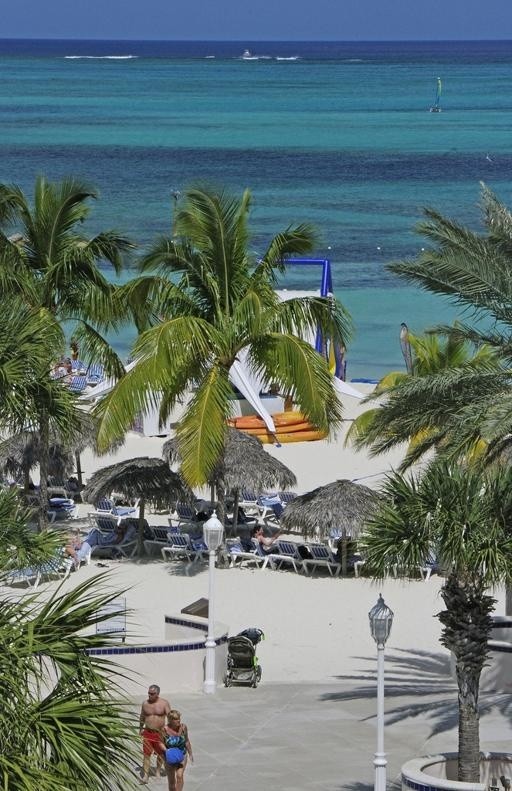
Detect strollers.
[227,627,266,689]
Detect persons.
[338,340,346,381]
[250,525,279,553]
[52,337,79,387]
[112,521,127,545]
[139,685,194,791]
[57,534,82,567]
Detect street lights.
[202,509,226,694]
[369,591,394,791]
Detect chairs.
[88,485,362,582]
[50,360,104,393]
[354,545,391,579]
[392,549,433,581]
[7,485,97,588]
